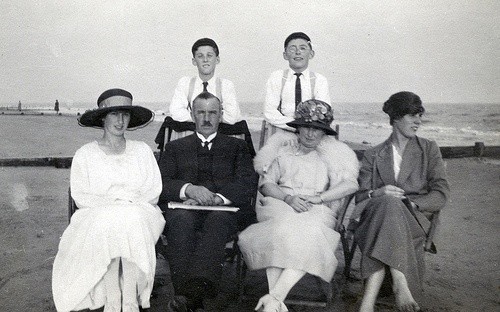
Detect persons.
[18,100,22,111]
[51,88,168,312]
[263,31,333,133]
[353,90,451,312]
[168,38,247,141]
[236,99,362,312]
[157,91,261,312]
[54,99,60,114]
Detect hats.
[78,88,155,131]
[285,99,339,137]
[383,91,425,125]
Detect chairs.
[162,116,251,300]
[258,119,350,307]
[343,146,447,307]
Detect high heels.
[254,294,282,312]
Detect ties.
[201,82,209,92]
[293,72,304,107]
[201,140,210,151]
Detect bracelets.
[368,189,374,198]
[283,193,292,202]
[319,195,324,203]
